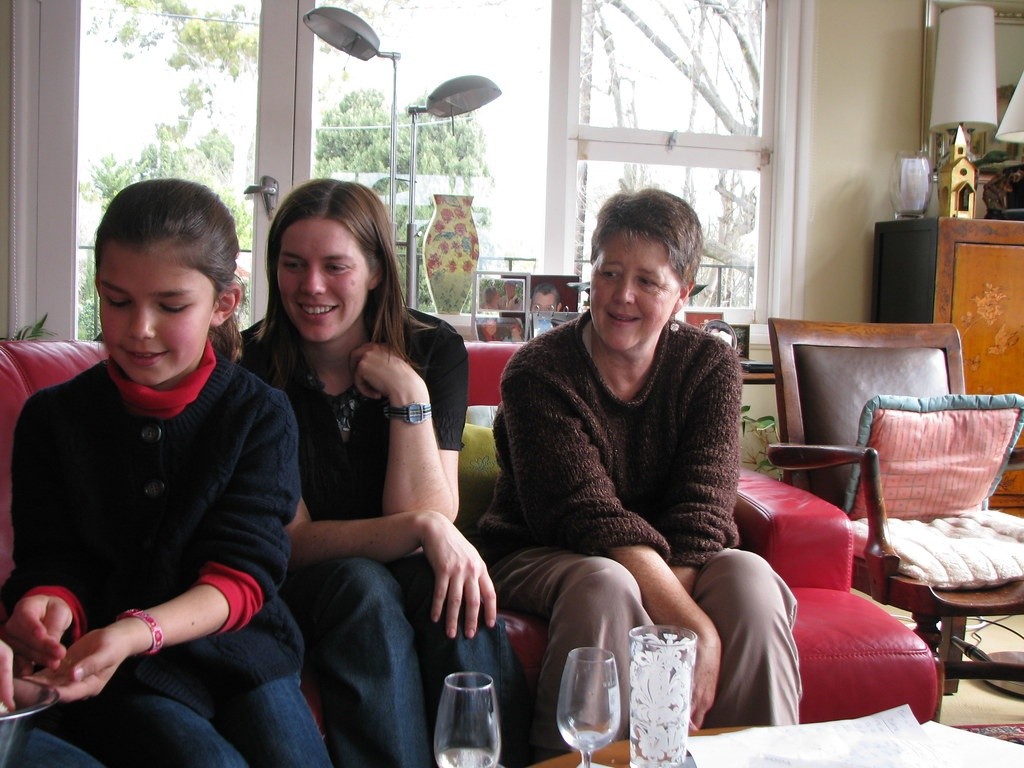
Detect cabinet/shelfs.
[869,218,1024,520]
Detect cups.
[888,151,932,221]
[628,624,698,768]
[434,671,502,768]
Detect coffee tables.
[529,726,745,768]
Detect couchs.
[0,341,947,724]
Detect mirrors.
[924,0,1024,182]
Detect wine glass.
[556,647,621,768]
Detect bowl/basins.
[0,675,60,768]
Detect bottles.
[423,192,480,315]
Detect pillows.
[845,393,1024,520]
[455,404,502,536]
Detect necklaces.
[302,348,357,442]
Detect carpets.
[950,723,1024,744]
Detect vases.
[422,194,479,315]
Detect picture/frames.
[684,311,725,330]
[471,271,532,342]
[971,84,1018,163]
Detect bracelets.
[116,608,163,656]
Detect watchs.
[383,403,431,424]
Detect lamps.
[929,6,997,221]
[995,70,1024,142]
[303,7,502,311]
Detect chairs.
[768,316,1024,694]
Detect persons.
[531,283,563,311]
[0,640,106,768]
[0,181,331,768]
[238,179,537,768]
[479,317,524,342]
[474,188,803,743]
[483,282,521,310]
